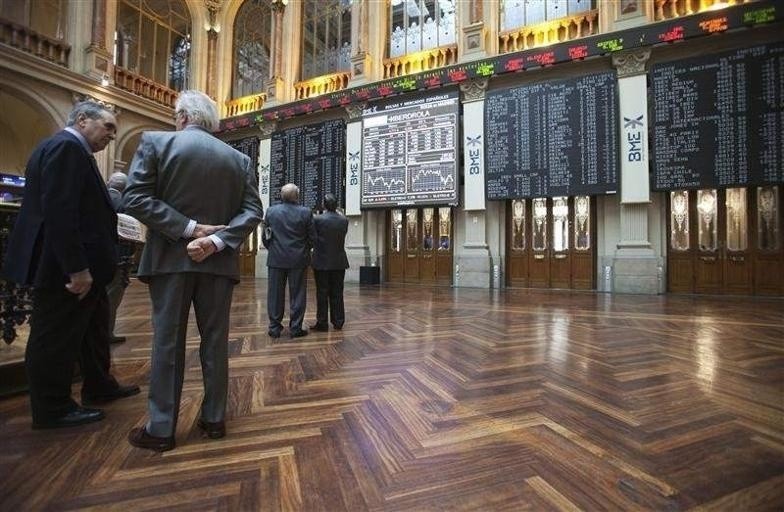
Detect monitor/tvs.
[0,172,28,202]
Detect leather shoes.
[268,331,280,338]
[197,414,226,440]
[81,385,141,404]
[32,407,105,429]
[107,335,128,344]
[128,426,176,452]
[290,330,307,338]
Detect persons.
[261,183,314,339]
[309,193,350,331]
[102,171,139,345]
[0,97,142,430]
[121,87,265,453]
